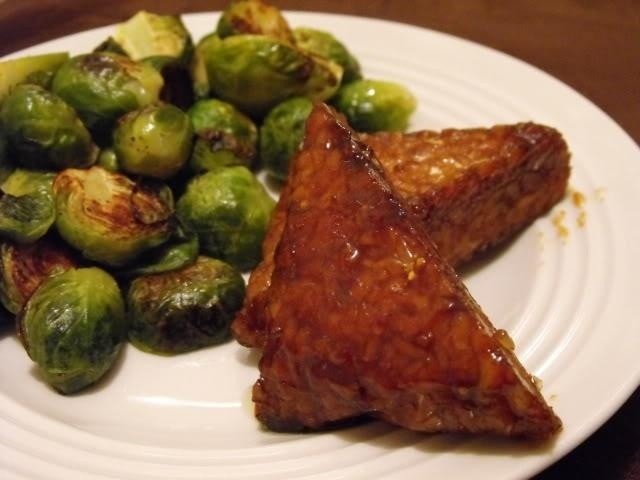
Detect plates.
[1,7,640,479]
